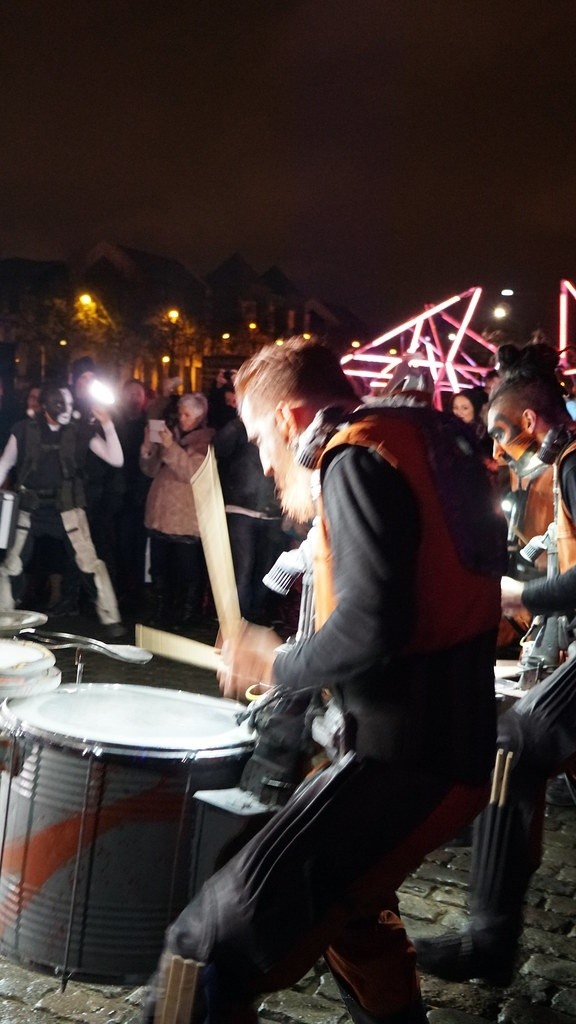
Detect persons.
[0,360,319,646]
[395,344,559,654]
[151,338,505,1024]
[412,368,576,985]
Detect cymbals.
[0,609,51,633]
[18,625,155,667]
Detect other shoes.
[108,623,128,637]
[270,600,287,625]
[412,920,514,989]
[252,614,275,630]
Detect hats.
[460,389,483,417]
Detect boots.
[146,594,175,628]
[172,583,202,633]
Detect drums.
[0,638,66,701]
[0,677,263,993]
